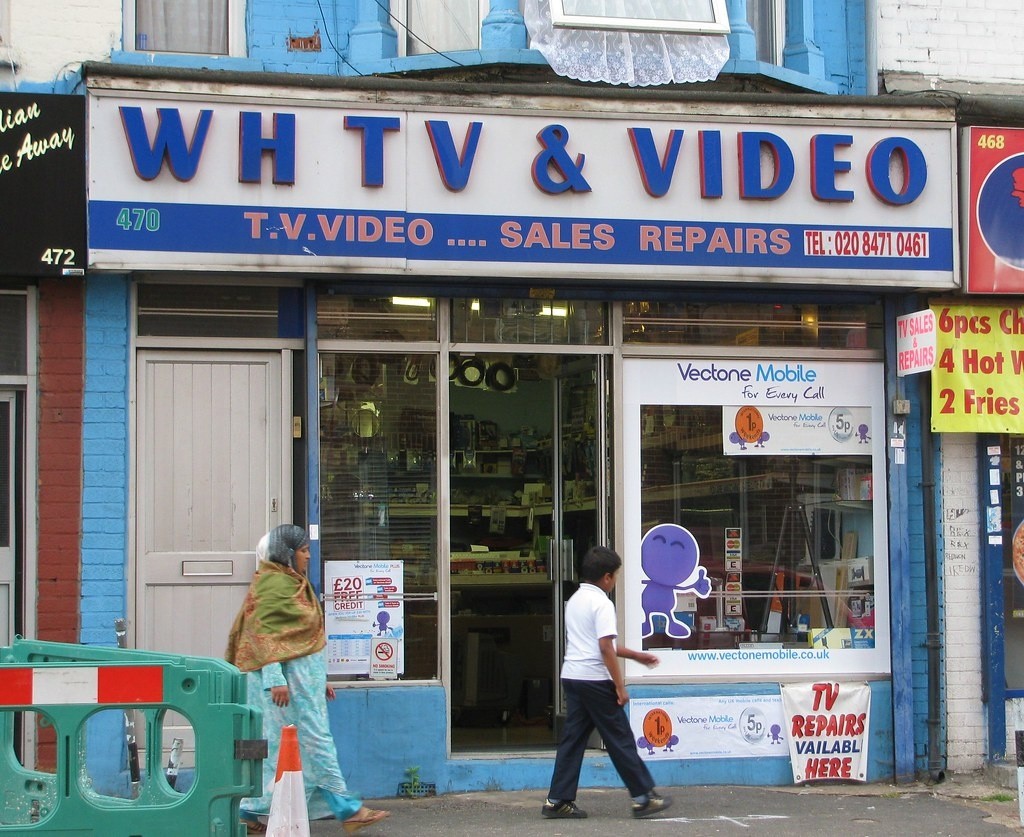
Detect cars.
[672,558,875,656]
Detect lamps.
[799,306,820,346]
[351,406,380,452]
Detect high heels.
[240,817,267,835]
[342,808,389,834]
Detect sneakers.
[541,796,587,818]
[632,792,674,817]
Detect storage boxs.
[847,556,873,587]
[699,615,746,649]
[836,468,873,503]
[808,627,876,649]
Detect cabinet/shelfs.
[321,425,836,728]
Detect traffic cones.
[265,724,311,837]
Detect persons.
[224,525,389,833]
[541,547,674,820]
